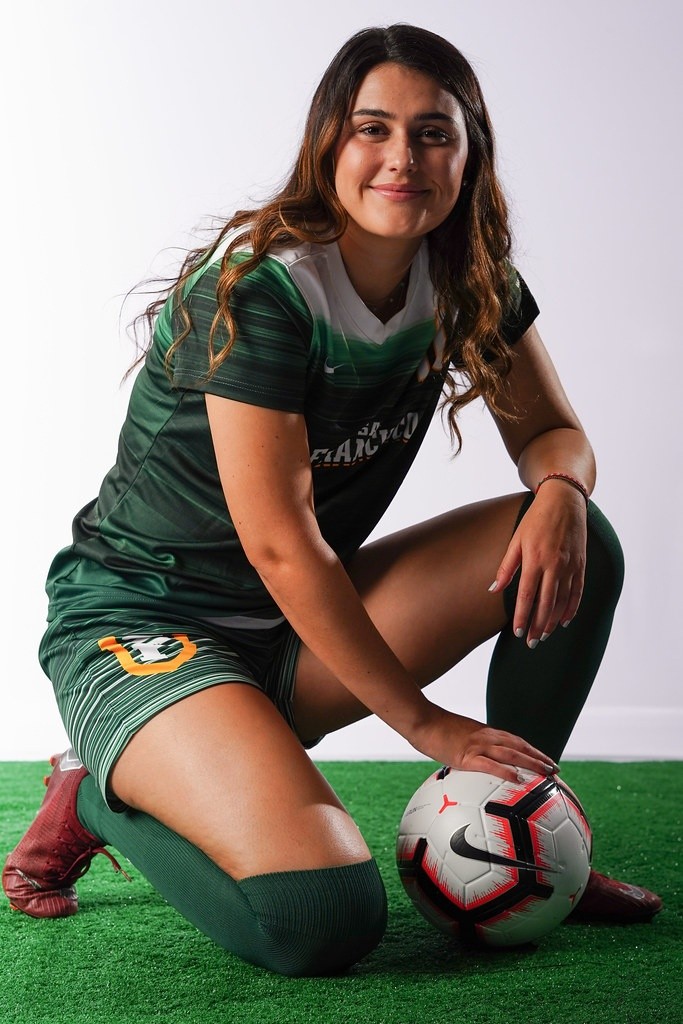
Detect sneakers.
[560,866,661,927]
[2,746,109,919]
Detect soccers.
[393,754,596,951]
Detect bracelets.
[535,473,590,510]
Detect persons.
[0,22,667,983]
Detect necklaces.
[358,282,406,312]
